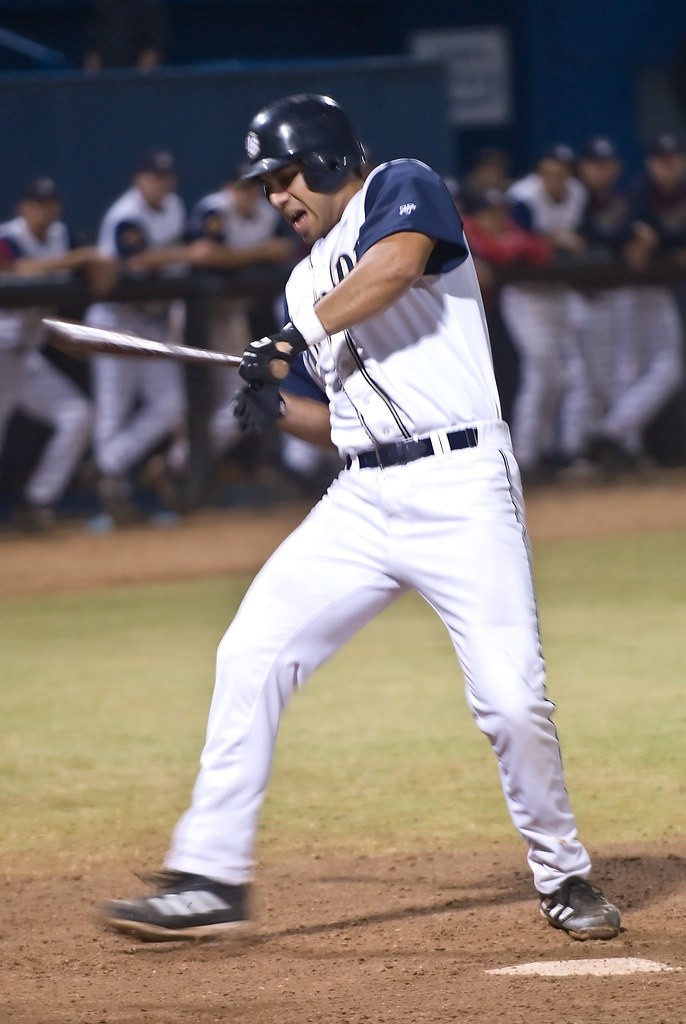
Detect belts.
[346,428,478,471]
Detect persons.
[83,0,170,70]
[186,161,280,458]
[0,175,101,531]
[455,132,686,470]
[84,152,216,495]
[103,93,620,942]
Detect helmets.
[240,94,366,192]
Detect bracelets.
[293,308,329,348]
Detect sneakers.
[101,870,254,941]
[539,877,621,940]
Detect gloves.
[237,321,308,391]
[232,383,286,434]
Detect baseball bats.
[39,317,290,381]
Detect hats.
[28,174,58,202]
[543,138,577,175]
[140,151,181,174]
[647,133,686,157]
[578,137,621,163]
